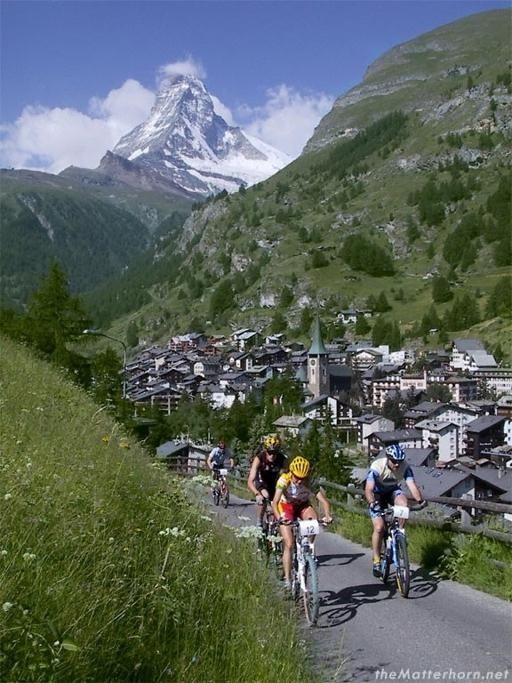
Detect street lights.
[82,329,127,417]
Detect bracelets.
[254,492,262,497]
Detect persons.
[269,455,333,592]
[245,436,293,534]
[206,440,235,498]
[366,444,427,578]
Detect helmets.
[290,456,311,479]
[264,433,280,451]
[386,444,406,460]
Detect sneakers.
[373,561,383,578]
[211,488,217,498]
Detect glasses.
[391,458,402,465]
[267,449,278,454]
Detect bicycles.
[210,467,235,509]
[370,499,427,599]
[251,497,334,630]
